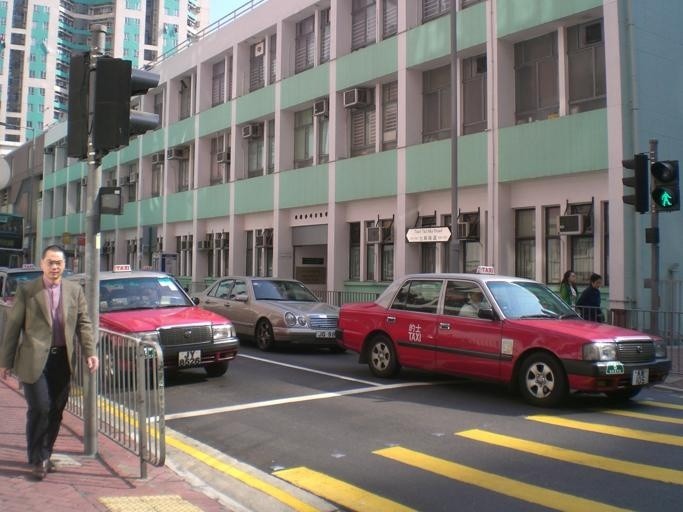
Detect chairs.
[444,287,470,313]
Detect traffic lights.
[127,68,160,135]
[649,160,678,210]
[621,156,640,207]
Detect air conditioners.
[104,123,262,186]
[447,221,479,242]
[254,234,273,248]
[343,88,371,110]
[558,214,593,238]
[313,100,328,120]
[102,236,227,257]
[365,227,393,246]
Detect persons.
[576,273,605,322]
[0,245,99,479]
[459,292,489,318]
[559,270,579,317]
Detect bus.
[0,212,25,297]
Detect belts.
[50,347,67,354]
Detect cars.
[64,266,237,386]
[338,266,670,408]
[0,263,44,306]
[189,276,346,352]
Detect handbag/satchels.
[593,314,605,321]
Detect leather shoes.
[32,456,55,478]
